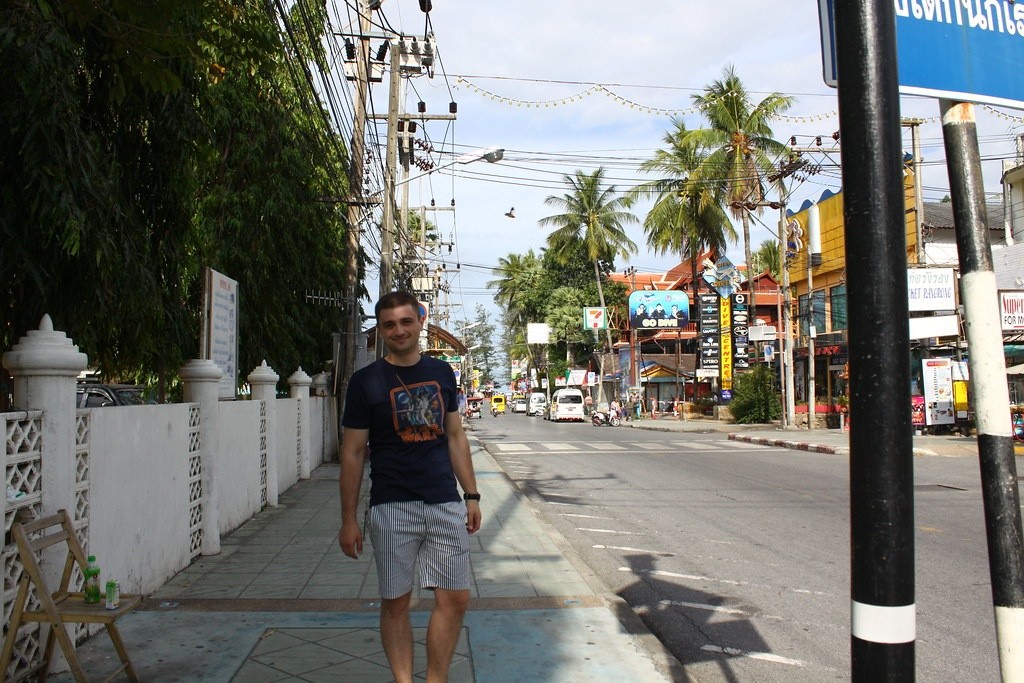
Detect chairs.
[0,509,141,683]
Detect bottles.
[82,555,101,604]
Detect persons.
[340,293,482,683]
[636,304,649,327]
[453,387,553,422]
[585,393,593,416]
[668,306,690,328]
[651,304,666,319]
[612,392,721,419]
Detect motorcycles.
[591,407,621,427]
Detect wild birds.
[504,207,516,219]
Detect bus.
[490,395,506,414]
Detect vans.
[549,387,584,422]
[525,392,546,416]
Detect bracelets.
[463,493,482,503]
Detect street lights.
[337,142,507,462]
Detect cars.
[510,398,526,412]
